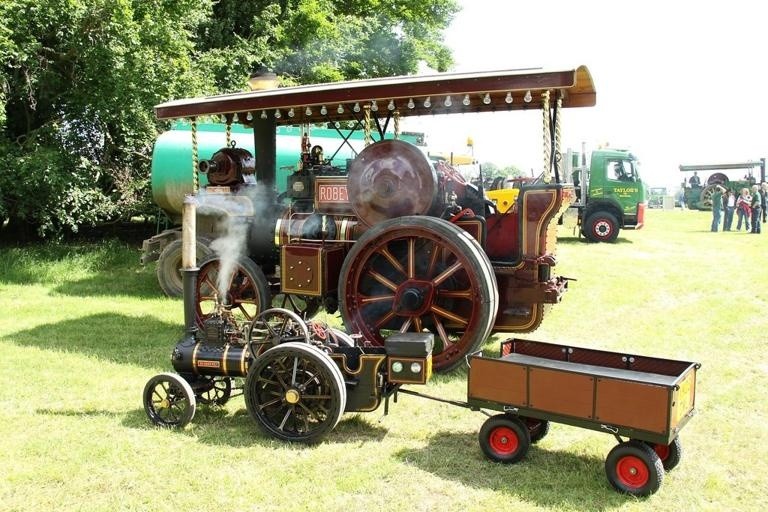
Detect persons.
[711,173,768,234]
[689,171,701,188]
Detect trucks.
[138,119,449,300]
[646,187,670,209]
[470,141,645,243]
